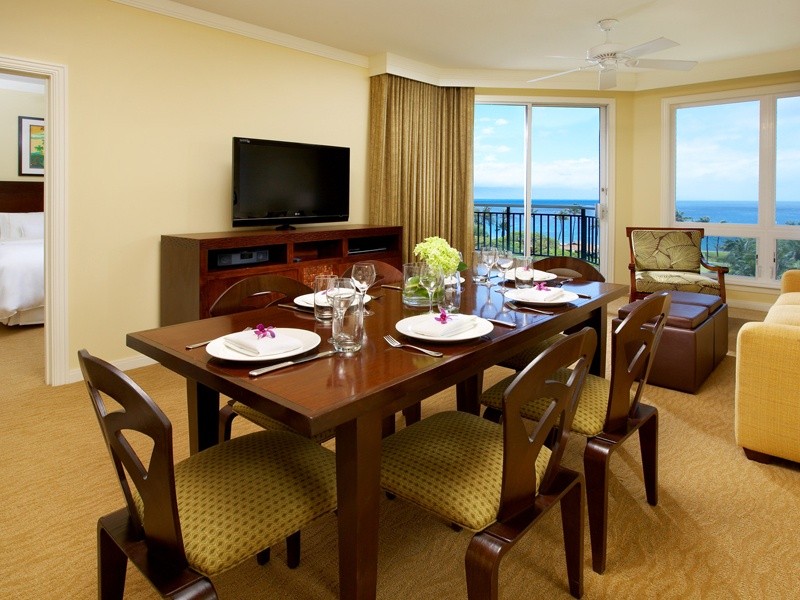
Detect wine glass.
[351,261,375,317]
[481,247,513,292]
[420,265,444,313]
[326,278,356,344]
[480,285,513,329]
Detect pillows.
[0,211,44,245]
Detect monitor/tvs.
[231,136,350,230]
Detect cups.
[437,271,461,314]
[472,250,487,282]
[515,256,534,290]
[402,262,445,306]
[332,294,363,352]
[314,274,340,323]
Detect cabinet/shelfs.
[160,223,405,326]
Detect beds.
[0,180,45,328]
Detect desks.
[126,273,630,600]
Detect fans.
[525,19,698,90]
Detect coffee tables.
[611,289,729,396]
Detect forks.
[383,335,443,357]
[506,301,554,315]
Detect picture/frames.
[18,116,45,177]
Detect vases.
[400,259,445,308]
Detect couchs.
[733,269,800,464]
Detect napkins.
[519,286,564,301]
[223,330,281,357]
[418,313,477,337]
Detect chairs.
[624,226,729,305]
[449,290,675,577]
[493,255,608,449]
[72,347,340,600]
[377,325,597,600]
[206,273,338,572]
[334,258,424,501]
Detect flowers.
[254,323,277,339]
[412,235,469,280]
[533,281,550,291]
[433,308,452,323]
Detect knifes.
[278,304,314,314]
[249,351,338,376]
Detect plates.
[497,269,558,281]
[504,288,579,306]
[293,288,371,307]
[205,327,321,362]
[408,275,465,285]
[394,314,494,341]
